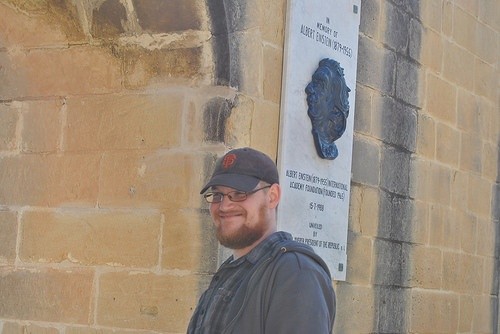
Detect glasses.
[204,184,273,203]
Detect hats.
[200,145,280,193]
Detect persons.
[186,147,336,334]
